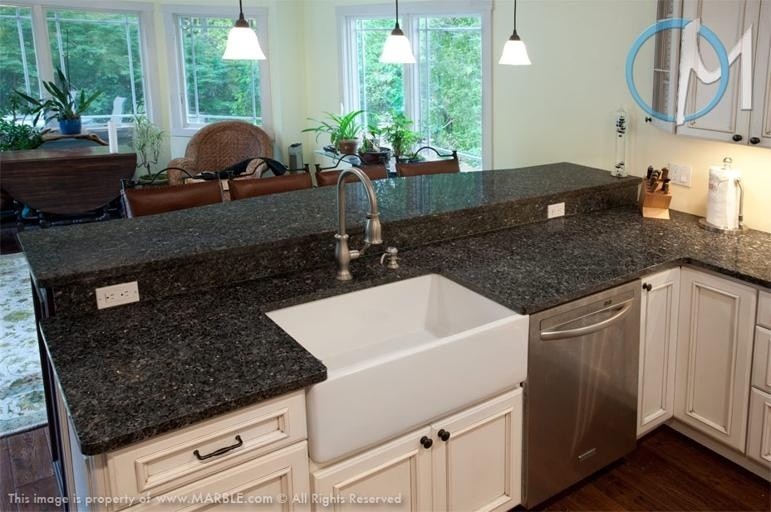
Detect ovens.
[521,279,641,508]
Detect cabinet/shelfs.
[0,144,137,231]
[89,389,311,512]
[636,265,771,481]
[645,0,771,148]
[313,384,525,512]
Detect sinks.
[265,273,530,464]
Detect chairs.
[167,121,273,200]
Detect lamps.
[497,1,532,66]
[221,1,266,62]
[378,0,417,65]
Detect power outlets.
[94,279,141,311]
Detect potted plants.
[358,112,391,166]
[300,108,365,155]
[125,114,169,186]
[11,67,105,135]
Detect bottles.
[609,109,629,179]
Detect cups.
[107,121,119,153]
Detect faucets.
[334,167,384,281]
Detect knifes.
[647,165,670,192]
